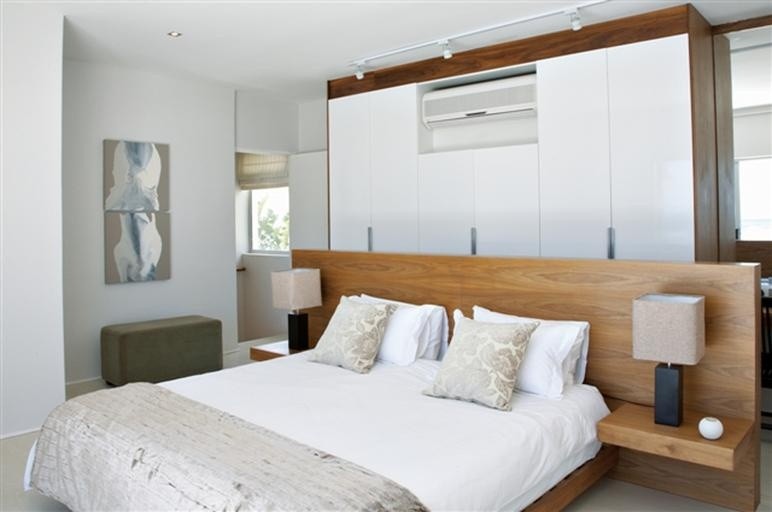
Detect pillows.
[308,292,591,412]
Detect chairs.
[758,296,772,432]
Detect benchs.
[100,315,223,388]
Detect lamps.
[270,267,322,352]
[350,1,582,79]
[630,292,707,427]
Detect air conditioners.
[422,71,537,130]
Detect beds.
[24,249,761,512]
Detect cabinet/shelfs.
[327,63,417,253]
[417,143,538,258]
[536,3,719,262]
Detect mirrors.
[713,23,772,243]
